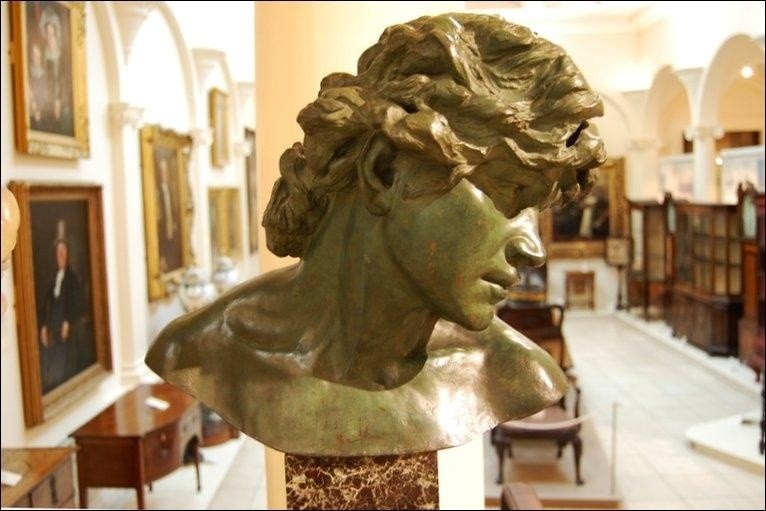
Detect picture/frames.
[9,178,114,429]
[138,121,199,303]
[8,1,90,162]
[539,158,633,261]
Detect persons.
[28,4,75,137]
[35,236,96,394]
[144,8,607,460]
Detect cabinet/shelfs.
[663,189,744,360]
[1,384,203,510]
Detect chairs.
[497,305,566,371]
[491,372,585,487]
